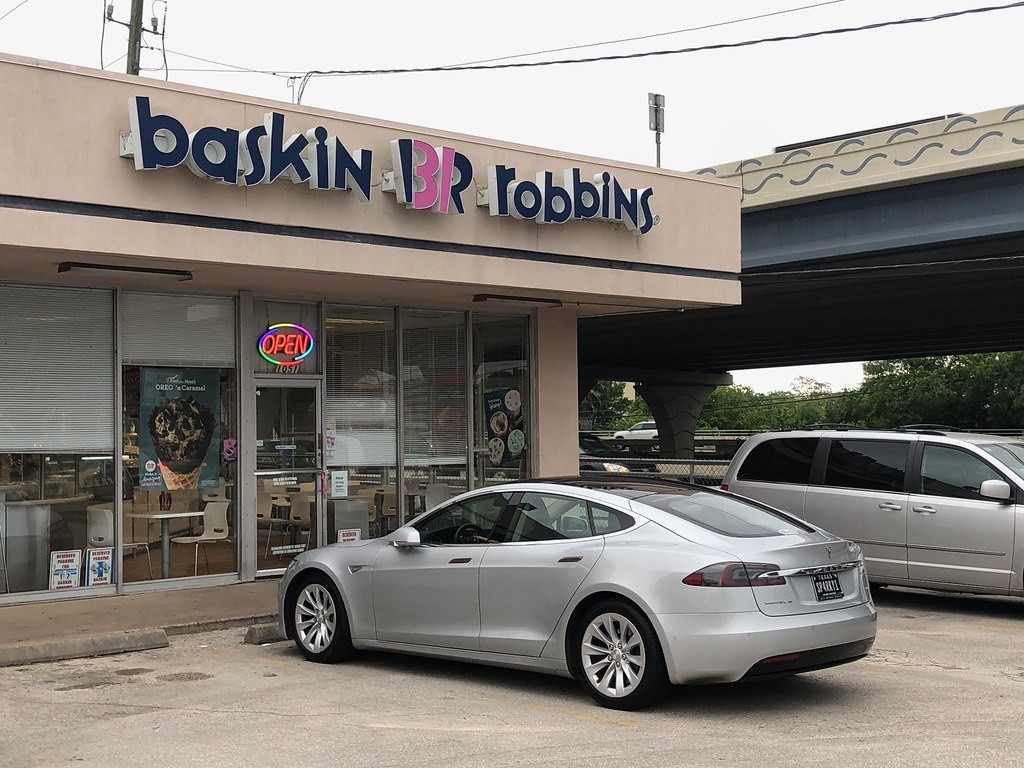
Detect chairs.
[169,502,232,577]
[518,495,558,542]
[81,508,154,580]
[256,479,450,562]
[203,477,232,505]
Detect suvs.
[720,423,1023,599]
[612,419,662,450]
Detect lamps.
[57,262,192,282]
[472,294,563,308]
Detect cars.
[485,432,661,481]
[280,474,879,713]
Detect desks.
[377,489,430,520]
[127,510,205,578]
[308,493,372,550]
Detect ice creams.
[149,395,215,491]
